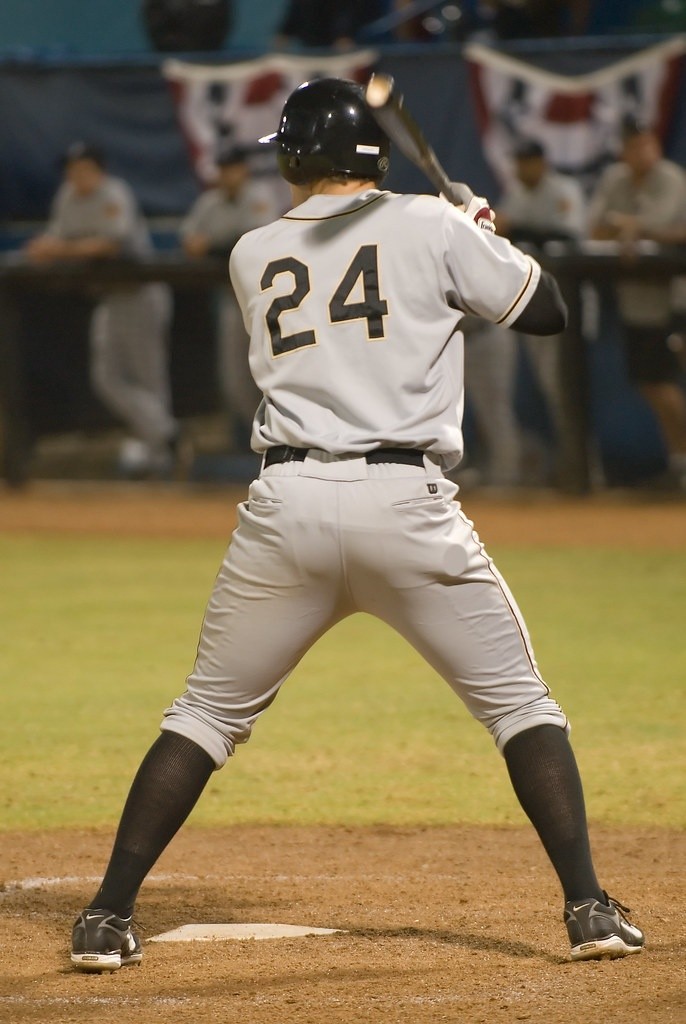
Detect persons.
[71,74,646,970]
[29,135,185,470]
[180,145,281,445]
[484,128,686,495]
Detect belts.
[264,446,424,467]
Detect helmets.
[258,77,389,182]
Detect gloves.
[440,181,496,233]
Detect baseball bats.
[366,70,463,208]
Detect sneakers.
[563,890,645,959]
[71,908,143,969]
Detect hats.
[514,142,540,160]
[214,145,244,167]
[624,114,650,136]
[59,144,106,170]
